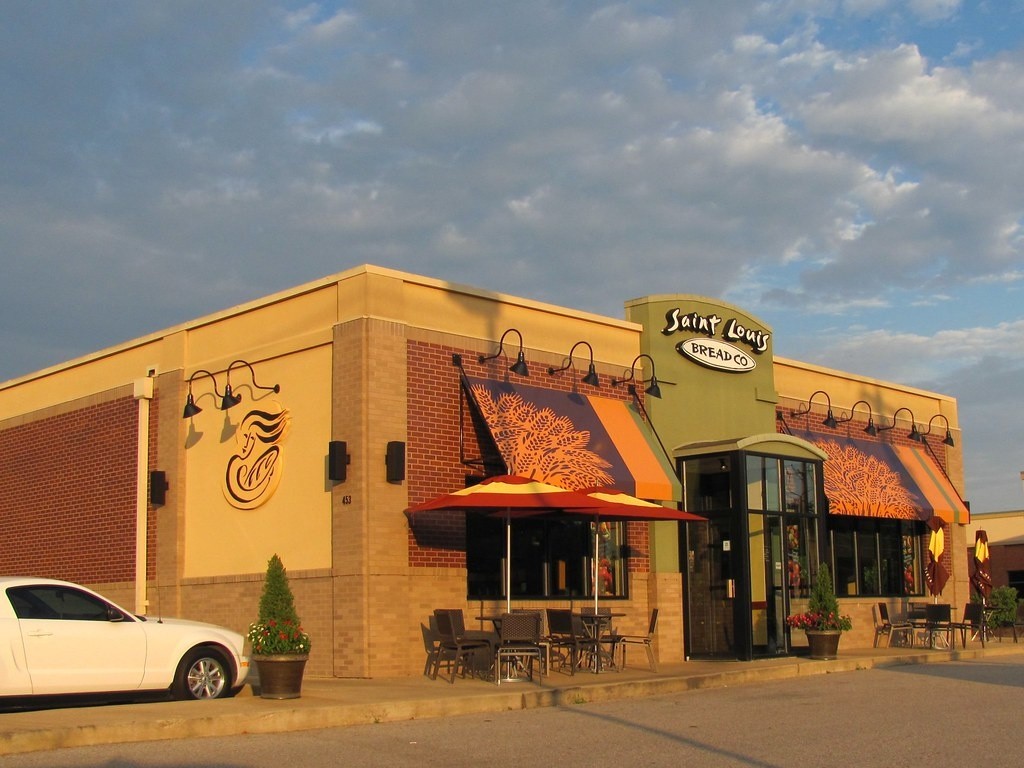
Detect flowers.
[786,563,853,632]
[247,553,313,654]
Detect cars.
[0,576,250,701]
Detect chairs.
[875,602,914,649]
[612,608,660,671]
[434,608,612,685]
[906,601,958,651]
[947,603,985,650]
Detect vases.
[805,630,842,660]
[251,654,309,698]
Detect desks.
[914,605,957,648]
[573,612,625,669]
[475,613,530,675]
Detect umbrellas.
[488,482,709,674]
[970,526,995,643]
[923,515,950,651]
[402,469,603,681]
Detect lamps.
[920,415,954,448]
[548,340,601,387]
[611,354,663,399]
[151,470,169,503]
[327,440,350,482]
[835,400,875,436]
[384,441,406,482]
[221,360,279,410]
[877,408,921,441]
[480,329,530,376]
[182,369,241,419]
[790,390,836,428]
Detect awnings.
[781,426,970,526]
[459,375,684,502]
